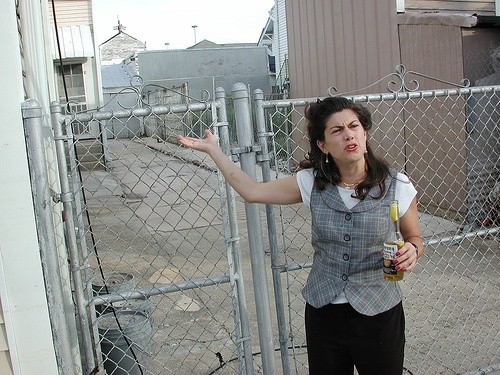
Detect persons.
[177,96,424,375]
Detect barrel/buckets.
[95,310,153,375]
[90,271,153,321]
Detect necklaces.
[341,175,366,190]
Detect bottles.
[383,200,405,281]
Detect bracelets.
[408,240,419,259]
